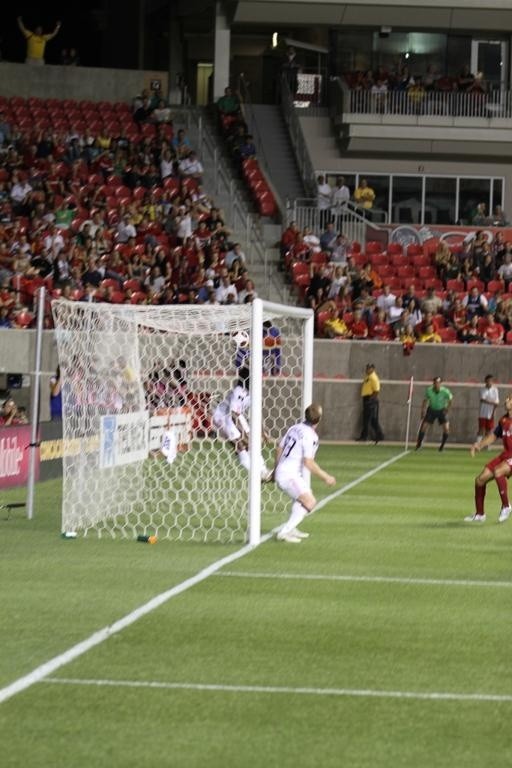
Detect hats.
[0,136,250,325]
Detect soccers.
[228,331,249,349]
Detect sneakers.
[372,436,385,442]
[463,512,486,522]
[497,503,511,522]
[276,526,310,544]
[355,436,368,442]
[416,439,422,448]
[439,443,444,451]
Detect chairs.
[278,235,512,343]
[214,94,279,216]
[1,95,199,304]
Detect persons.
[354,362,386,441]
[414,375,454,451]
[471,374,499,456]
[16,15,61,65]
[464,397,512,524]
[273,403,336,544]
[213,369,275,482]
